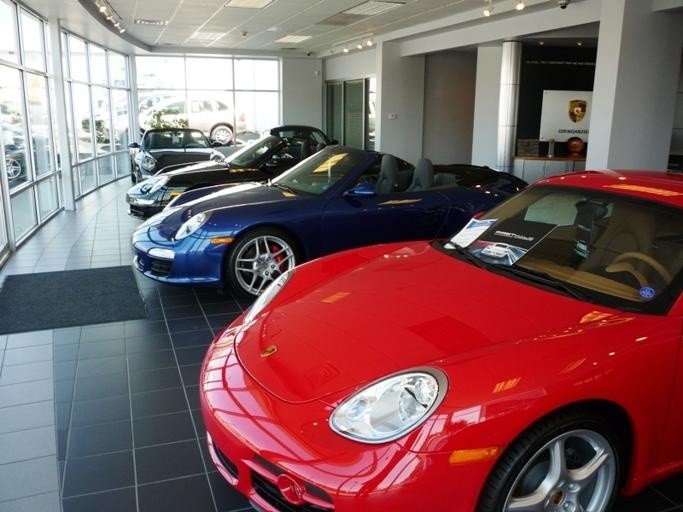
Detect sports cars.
[199,168,683,512]
[126,124,340,219]
[128,145,528,301]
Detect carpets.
[0,264,151,335]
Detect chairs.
[401,156,432,192]
[579,200,655,287]
[374,150,398,196]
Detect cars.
[0,89,250,184]
